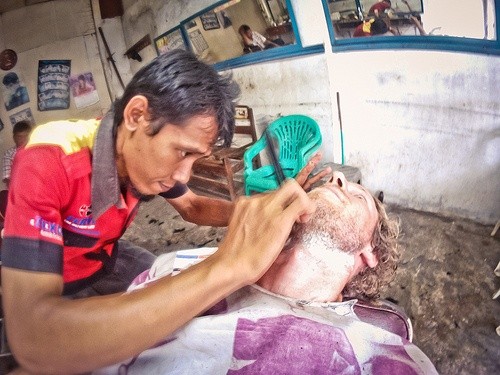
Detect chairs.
[243,114,322,196]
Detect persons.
[74,72,94,97]
[2,72,30,111]
[2,119,33,188]
[1,47,333,375]
[353,0,431,37]
[238,24,281,53]
[7,170,441,375]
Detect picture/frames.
[154,24,187,57]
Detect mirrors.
[180,0,302,65]
[322,0,500,55]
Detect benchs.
[187,105,263,200]
